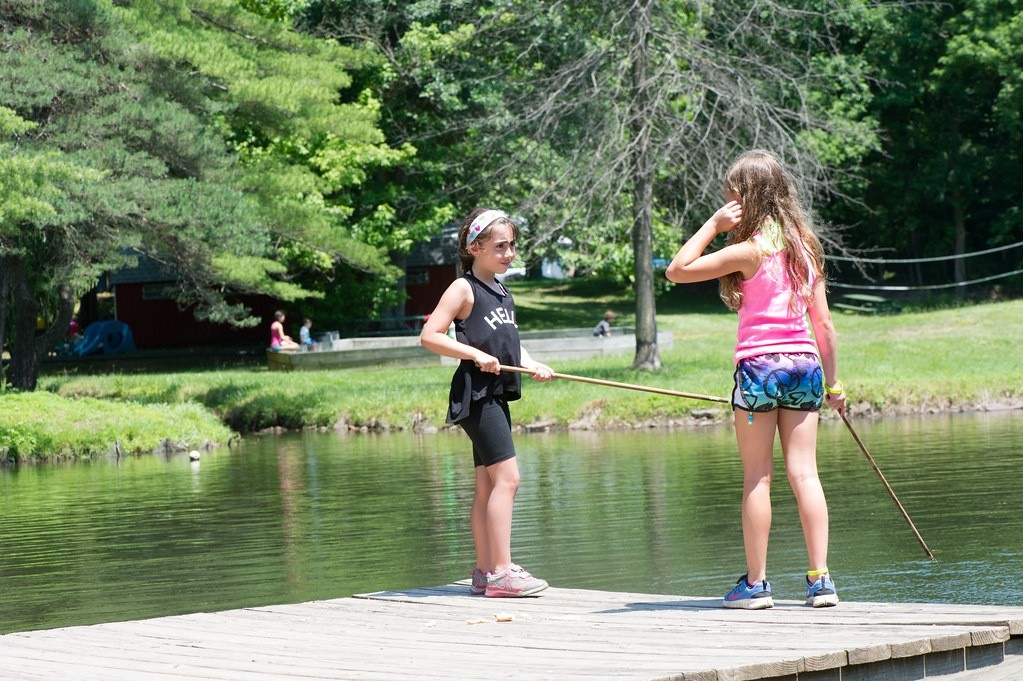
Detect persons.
[300,318,319,352]
[419,206,554,597]
[594,311,617,338]
[665,148,845,611]
[271,310,300,352]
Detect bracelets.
[824,379,843,395]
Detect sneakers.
[722,574,773,609]
[470,570,490,594]
[484,564,548,597]
[805,574,839,607]
